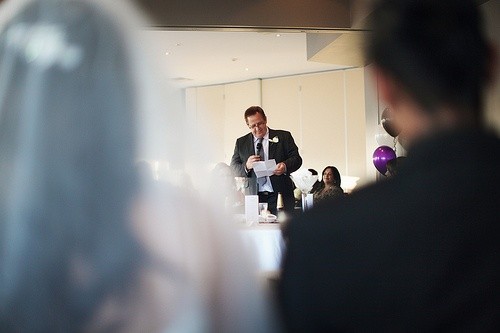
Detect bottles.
[276,192,284,219]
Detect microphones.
[256,143,262,155]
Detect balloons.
[372,146,396,176]
[381,106,405,136]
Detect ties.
[257,138,266,186]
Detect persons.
[211,162,246,213]
[230,106,302,218]
[283,0,500,332]
[294,166,344,207]
[0,0,275,333]
